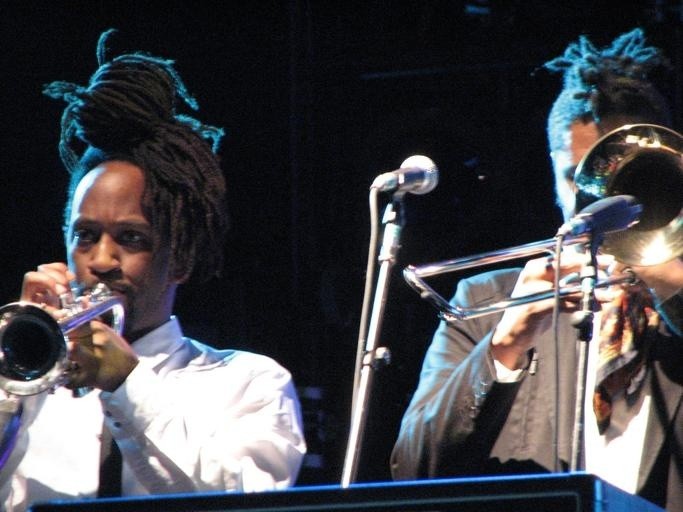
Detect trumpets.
[0,282,126,397]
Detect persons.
[389,26,683,509]
[0,27,309,511]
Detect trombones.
[404,124,683,321]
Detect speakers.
[28,472,662,510]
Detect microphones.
[374,155,439,195]
[557,192,643,237]
